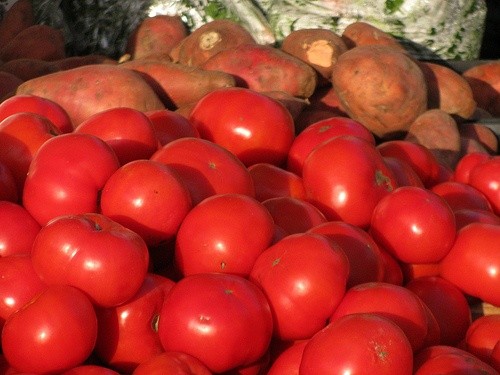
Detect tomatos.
[0,89,500,375]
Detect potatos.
[0,1,500,163]
